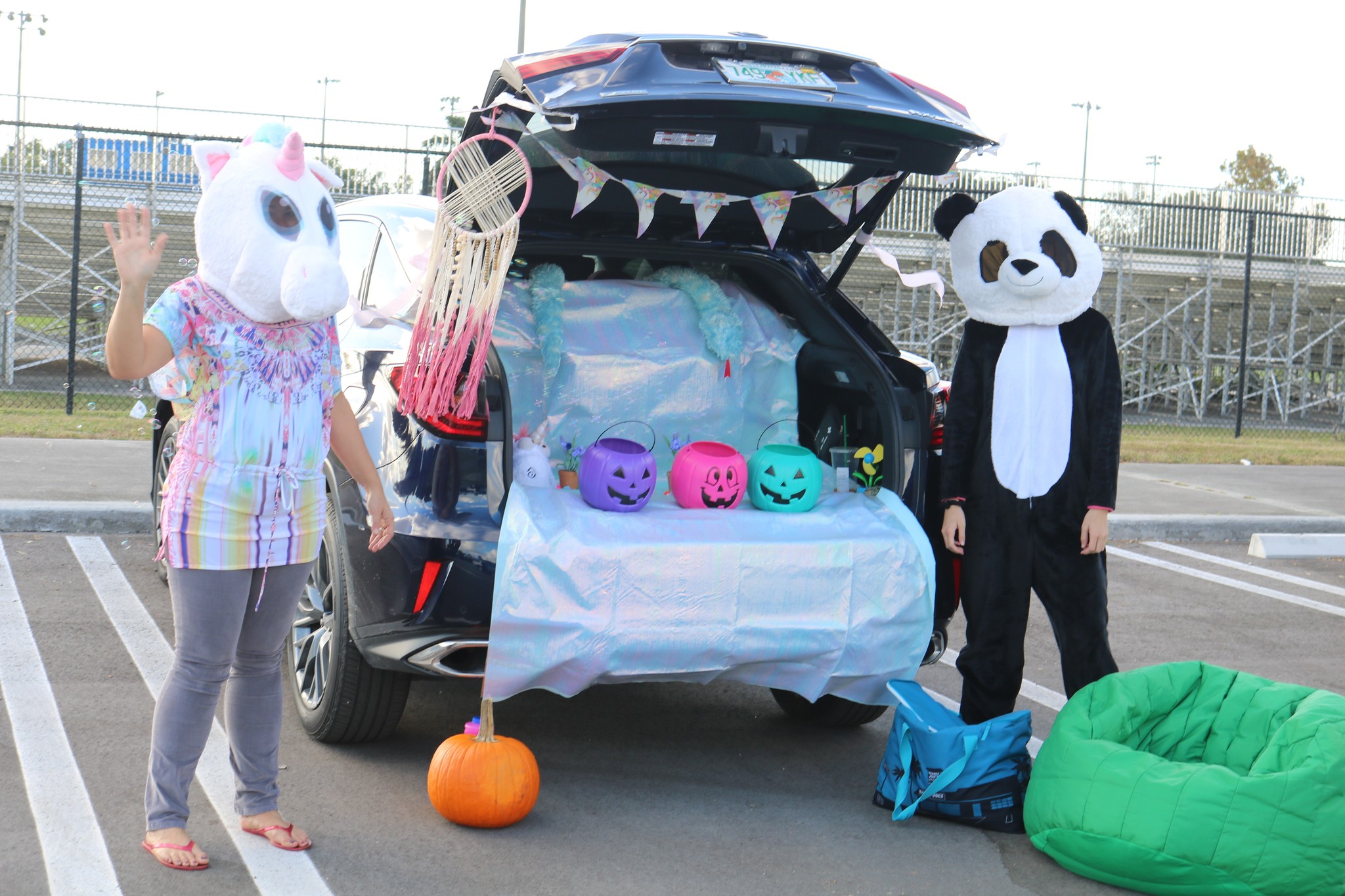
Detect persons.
[102,124,399,871]
[927,185,1117,723]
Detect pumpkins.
[426,701,540,829]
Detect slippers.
[140,836,209,869]
[240,814,309,851]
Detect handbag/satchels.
[871,679,1031,834]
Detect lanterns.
[744,419,823,512]
[579,420,659,512]
[671,441,747,509]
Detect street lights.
[1070,101,1102,211]
[441,95,460,192]
[0,9,49,223]
[317,76,340,165]
[1026,162,1041,176]
[1145,155,1162,203]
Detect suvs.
[152,30,962,747]
